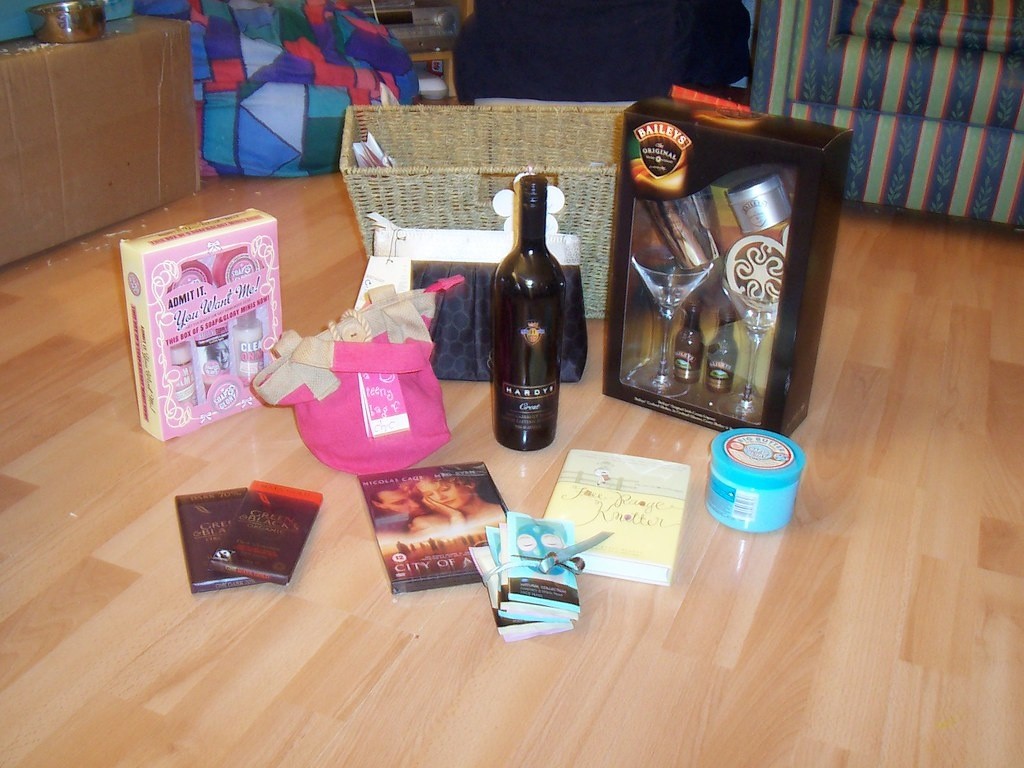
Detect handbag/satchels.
[366,206,589,383]
[251,275,463,476]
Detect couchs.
[134,0,420,178]
[749,0,1024,233]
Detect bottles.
[674,303,705,385]
[705,308,738,395]
[489,176,566,452]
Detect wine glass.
[715,275,779,425]
[632,247,714,399]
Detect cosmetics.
[706,429,806,532]
[120,201,283,442]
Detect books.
[545,449,691,586]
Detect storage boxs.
[602,99,854,439]
[0,8,200,267]
[118,208,282,442]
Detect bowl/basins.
[25,0,107,44]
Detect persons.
[372,468,505,539]
[207,340,229,369]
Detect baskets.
[340,105,624,318]
